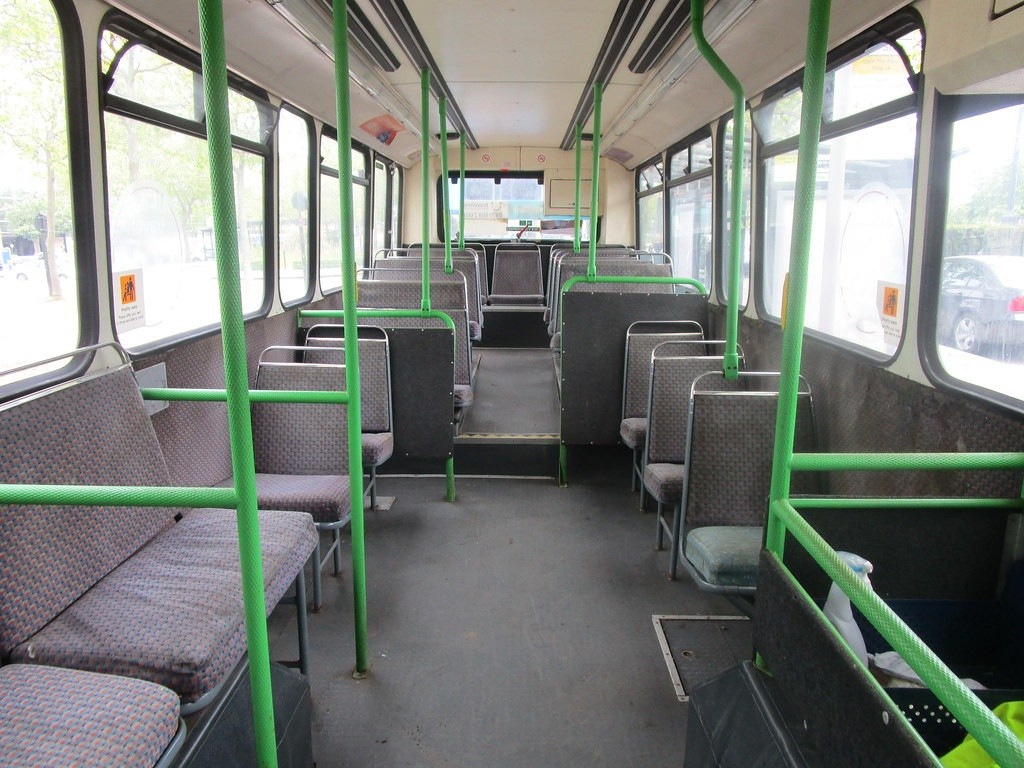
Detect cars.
[934,253,1024,358]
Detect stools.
[0,662,186,768]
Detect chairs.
[678,370,821,596]
[642,340,748,581]
[488,242,545,304]
[214,346,351,615]
[303,323,395,511]
[620,321,707,510]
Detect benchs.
[352,241,489,409]
[0,342,311,713]
[544,244,674,346]
